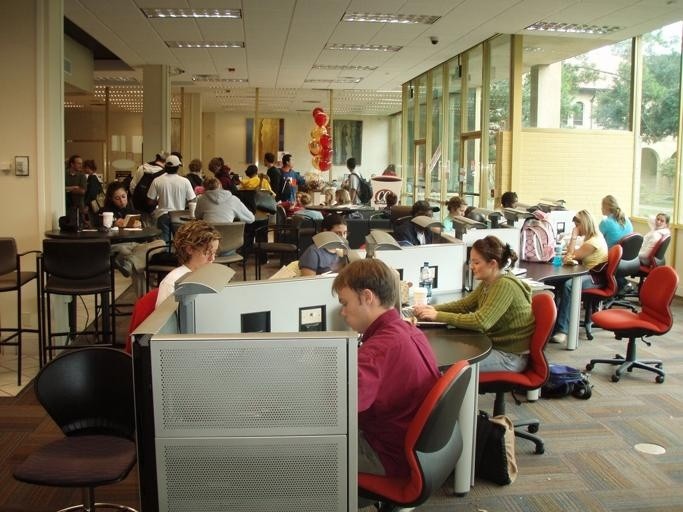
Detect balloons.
[308,107,333,171]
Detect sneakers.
[553,332,566,344]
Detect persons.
[153,221,223,311]
[63,151,312,280]
[599,195,634,248]
[552,209,609,343]
[638,212,670,267]
[412,235,535,374]
[297,155,519,277]
[331,257,442,478]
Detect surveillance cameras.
[430,35,439,45]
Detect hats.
[164,155,181,168]
[411,199,440,215]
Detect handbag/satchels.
[254,189,276,213]
[476,410,517,484]
[92,193,105,213]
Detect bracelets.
[571,236,577,240]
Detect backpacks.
[542,366,592,400]
[347,173,372,204]
[133,172,165,212]
[272,176,291,202]
[521,211,557,262]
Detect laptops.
[388,267,449,326]
[234,189,257,221]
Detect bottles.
[419,262,432,304]
[554,241,561,266]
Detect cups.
[189,202,196,218]
[413,288,427,307]
[102,212,114,228]
